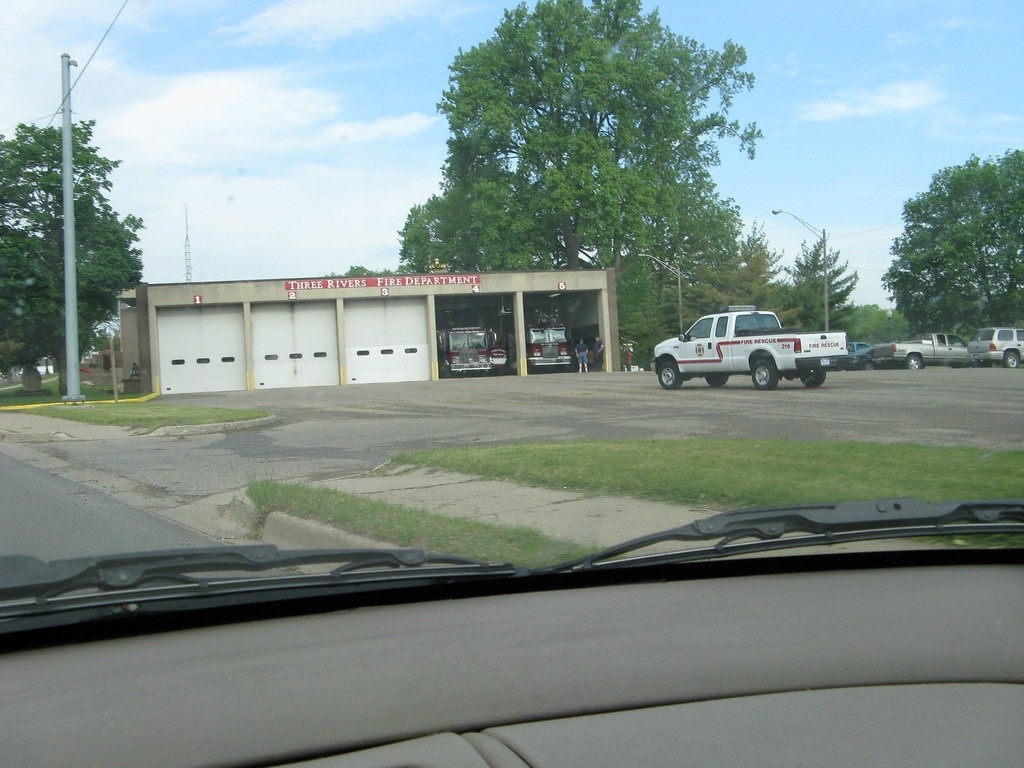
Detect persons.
[593,336,604,372]
[575,338,588,373]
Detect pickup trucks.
[651,304,849,391]
[871,332,971,370]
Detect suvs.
[966,326,1024,368]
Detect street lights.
[773,208,829,334]
[637,253,684,336]
[886,310,893,343]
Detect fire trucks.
[526,323,579,375]
[437,326,508,377]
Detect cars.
[847,347,874,372]
[825,341,871,372]
